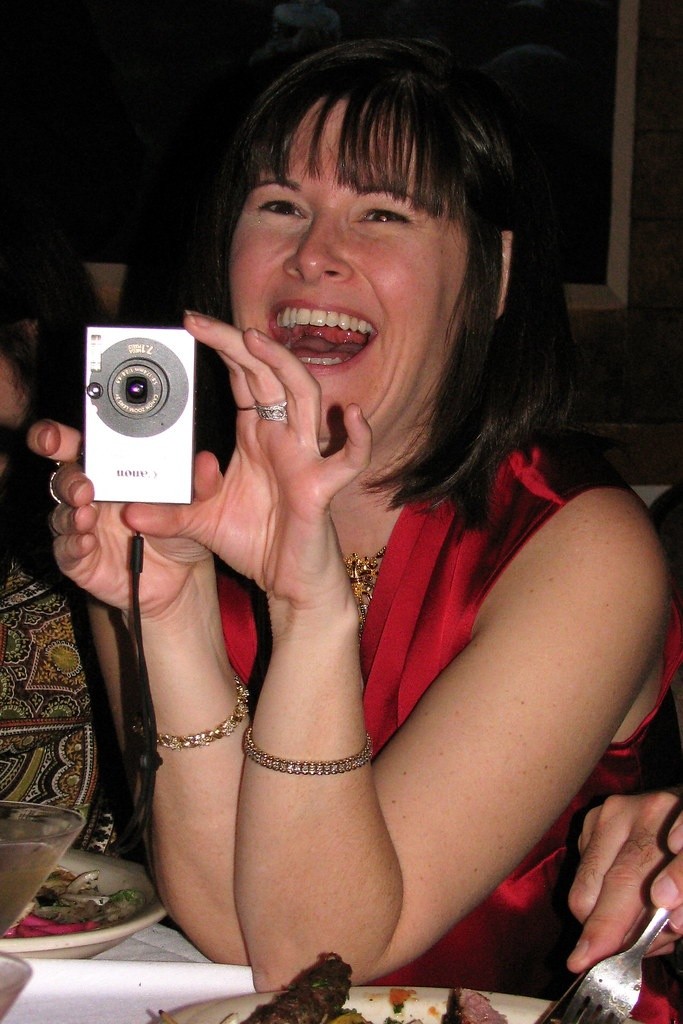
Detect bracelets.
[242,722,373,776]
[131,675,250,752]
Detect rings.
[236,405,256,411]
[50,461,65,505]
[255,400,288,422]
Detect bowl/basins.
[0,848,167,961]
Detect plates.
[156,986,643,1024]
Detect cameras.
[83,324,194,504]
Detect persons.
[565,489,683,975]
[0,213,116,937]
[27,36,683,992]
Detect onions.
[60,869,110,905]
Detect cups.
[0,952,32,1024]
[0,802,86,942]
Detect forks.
[537,907,669,1024]
